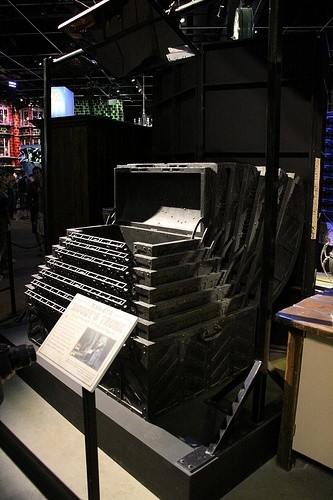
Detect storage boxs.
[24,161,299,420]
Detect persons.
[0,167,45,281]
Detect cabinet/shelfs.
[29,116,153,254]
[0,101,44,171]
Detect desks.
[275,290,333,471]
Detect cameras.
[0,343,37,379]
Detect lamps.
[231,8,256,41]
